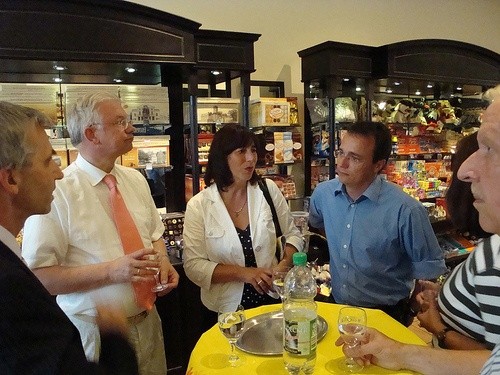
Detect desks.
[186,301,428,375]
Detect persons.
[306,121,448,329]
[182,123,304,328]
[335,85,500,375]
[22,89,180,375]
[0,100,139,375]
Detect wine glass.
[338,307,367,372]
[271,266,291,311]
[420,273,440,312]
[217,303,247,367]
[140,250,168,293]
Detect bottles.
[282,251,318,374]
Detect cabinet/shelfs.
[0,0,500,375]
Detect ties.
[102,174,157,313]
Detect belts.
[128,310,148,328]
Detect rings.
[257,280,262,284]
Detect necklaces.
[218,184,247,217]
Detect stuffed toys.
[331,92,465,130]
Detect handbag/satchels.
[277,235,287,261]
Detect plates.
[233,309,328,356]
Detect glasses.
[333,149,373,164]
[93,115,132,129]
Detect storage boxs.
[249,101,290,127]
[254,132,302,163]
[159,212,186,264]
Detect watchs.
[437,327,453,346]
